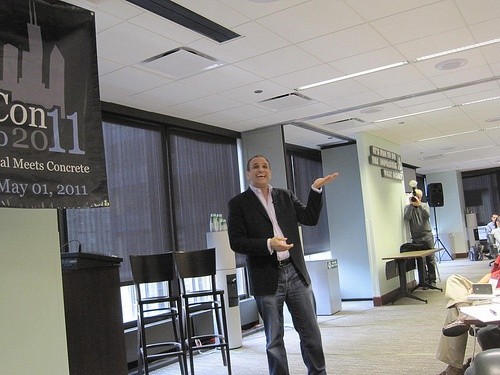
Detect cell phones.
[473,284,492,294]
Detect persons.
[485,213,500,253]
[404,189,437,289]
[228,155,339,375]
[436,255,500,375]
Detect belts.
[278,257,293,267]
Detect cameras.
[409,180,417,202]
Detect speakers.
[427,183,444,207]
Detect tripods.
[433,207,454,264]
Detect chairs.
[487,234,499,266]
[174,247,232,375]
[129,251,188,375]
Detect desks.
[382,248,444,304]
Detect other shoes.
[430,282,436,288]
[423,286,429,290]
[442,316,482,337]
[438,371,464,375]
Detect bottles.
[210,213,227,232]
